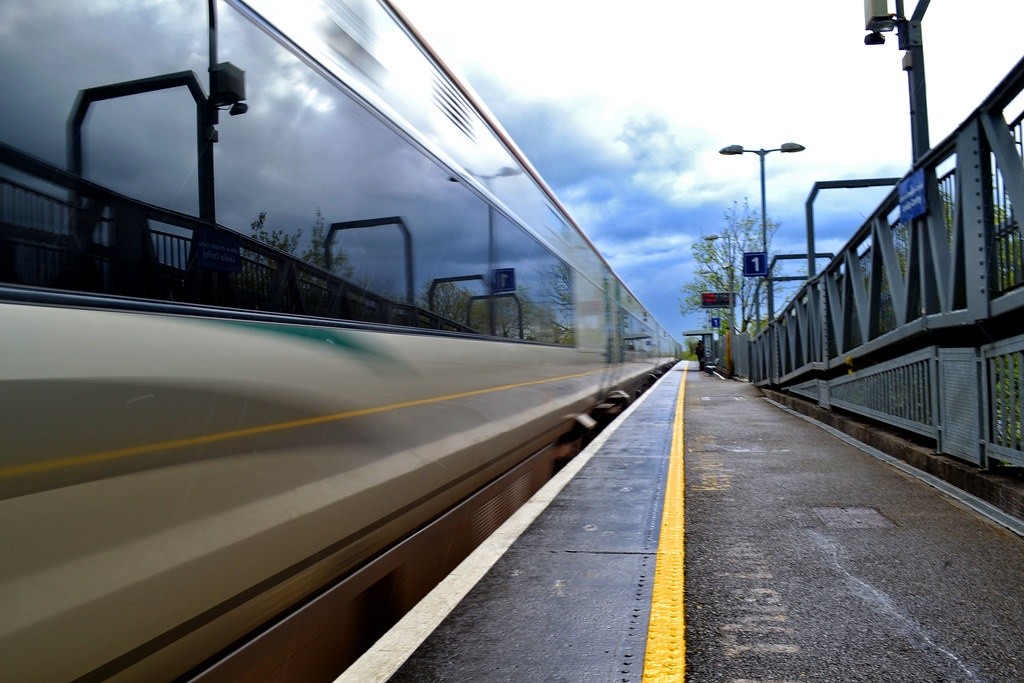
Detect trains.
[0,0,682,683]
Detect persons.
[695,340,704,371]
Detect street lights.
[719,143,805,324]
[705,235,735,362]
